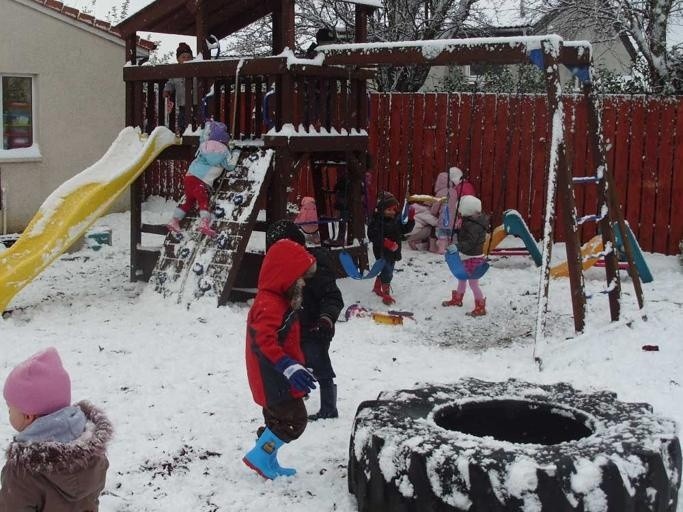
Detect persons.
[264,219,344,420]
[306,28,336,129]
[405,166,489,316]
[293,196,320,245]
[162,42,204,132]
[368,190,402,305]
[0,346,111,512]
[241,241,318,480]
[169,121,241,234]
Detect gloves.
[445,242,457,255]
[277,357,318,394]
[163,90,171,98]
[407,206,417,220]
[384,238,399,251]
[232,146,242,158]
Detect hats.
[449,166,464,185]
[2,345,72,416]
[457,194,482,219]
[208,120,231,145]
[266,219,307,252]
[177,42,192,59]
[376,191,400,215]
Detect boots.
[371,275,382,297]
[408,239,419,251]
[381,283,396,306]
[242,427,298,483]
[199,209,217,238]
[437,239,448,253]
[307,385,339,421]
[429,237,439,253]
[464,298,487,317]
[167,207,187,235]
[442,290,464,307]
